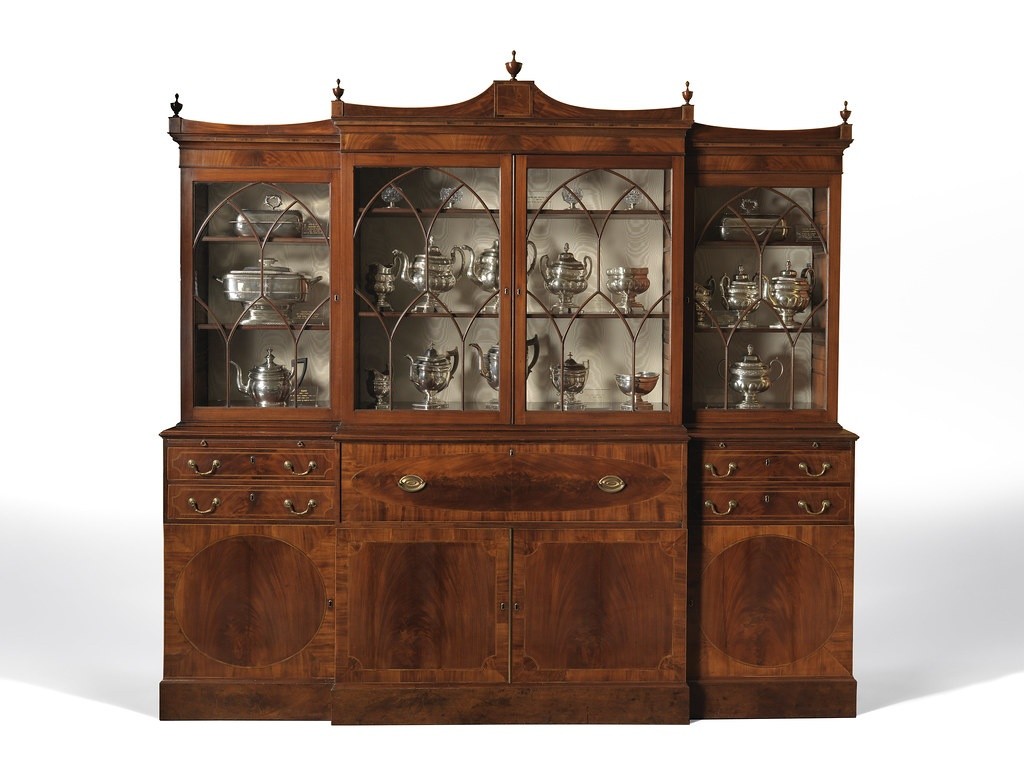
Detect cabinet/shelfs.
[156,50,860,726]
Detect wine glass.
[623,188,643,210]
[607,266,651,313]
[365,256,402,313]
[614,371,660,410]
[440,187,464,209]
[562,188,583,210]
[364,362,394,409]
[381,187,404,209]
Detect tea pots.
[230,348,308,408]
[461,241,536,313]
[403,342,459,409]
[469,334,540,408]
[392,235,466,312]
[753,259,815,328]
